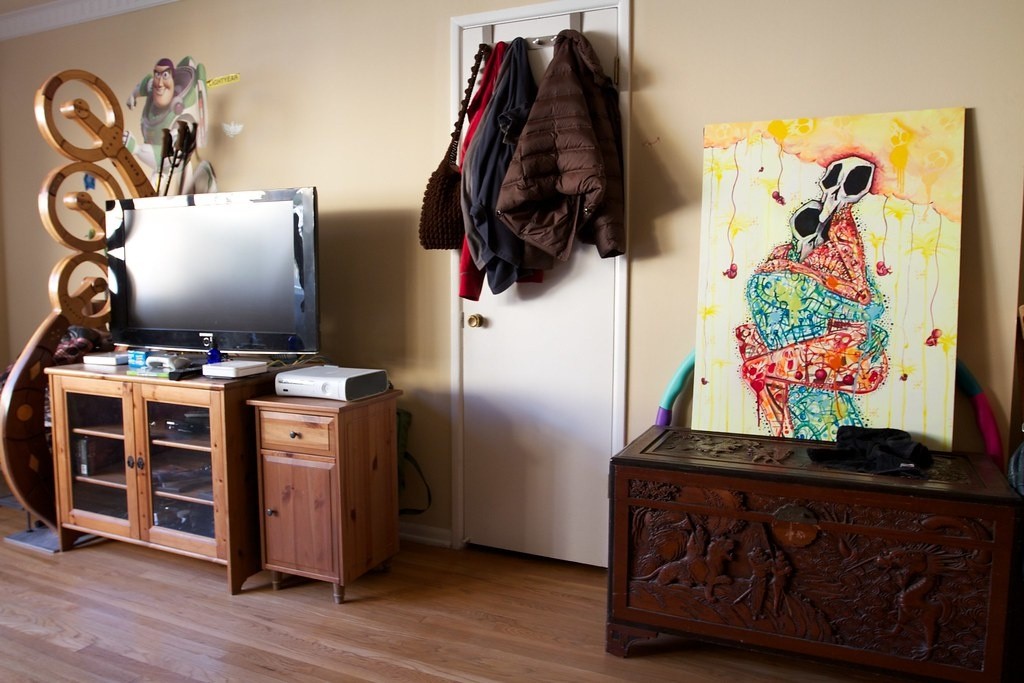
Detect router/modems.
[202,360,267,378]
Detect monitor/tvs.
[106,186,320,367]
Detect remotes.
[169,366,202,381]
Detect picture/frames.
[690,105,967,457]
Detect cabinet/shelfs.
[43,351,327,595]
[604,426,1024,683]
[245,390,403,604]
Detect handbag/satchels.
[418,149,465,249]
